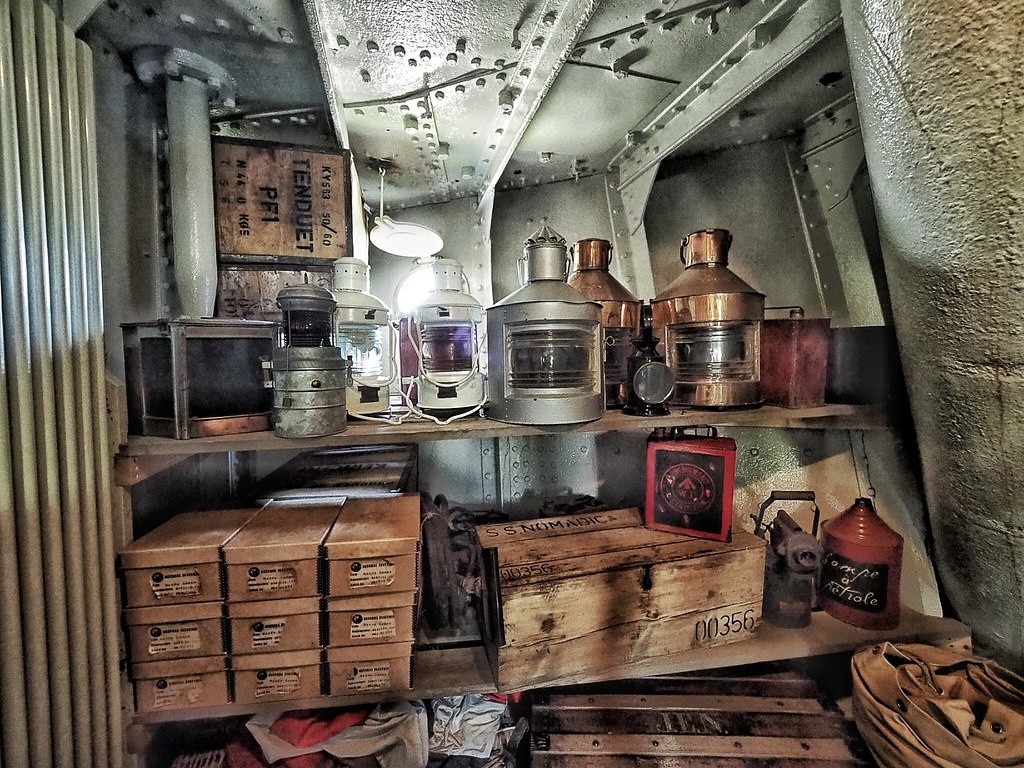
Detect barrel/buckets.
[762,305,832,407]
[827,326,893,405]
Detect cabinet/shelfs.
[118,404,973,755]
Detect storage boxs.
[130,655,229,714]
[231,649,323,705]
[221,496,348,603]
[326,641,416,697]
[122,601,227,664]
[326,591,416,649]
[324,491,421,598]
[474,506,768,695]
[118,508,260,609]
[225,595,324,656]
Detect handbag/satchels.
[852,641,1024,768]
